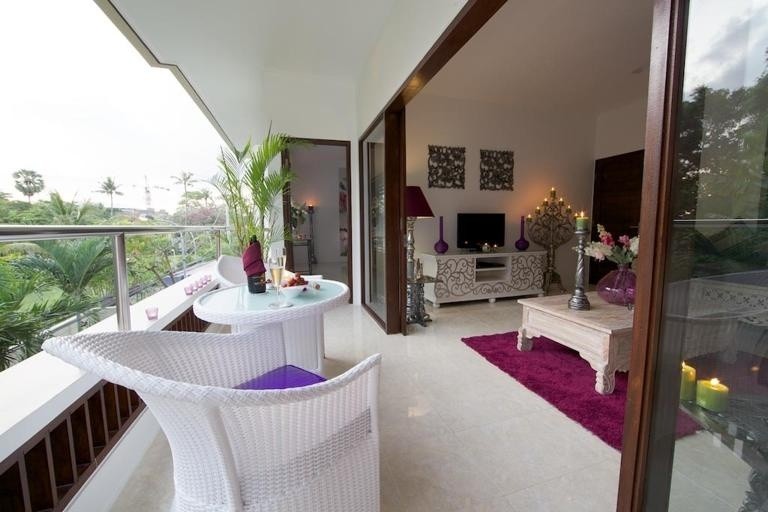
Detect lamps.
[407,186,435,221]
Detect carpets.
[460,329,768,458]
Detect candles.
[535,206,540,214]
[526,214,532,221]
[542,198,548,205]
[565,205,572,213]
[679,360,696,401]
[576,210,589,230]
[696,377,729,413]
[558,197,563,205]
[550,187,556,198]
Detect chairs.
[214,254,323,289]
[42,322,381,512]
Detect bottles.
[248,234,266,294]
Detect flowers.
[572,223,640,264]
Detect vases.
[515,216,529,252]
[434,215,449,254]
[596,264,637,307]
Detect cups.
[269,258,284,298]
[276,248,286,267]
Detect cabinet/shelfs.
[418,248,548,308]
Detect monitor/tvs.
[456,212,505,253]
